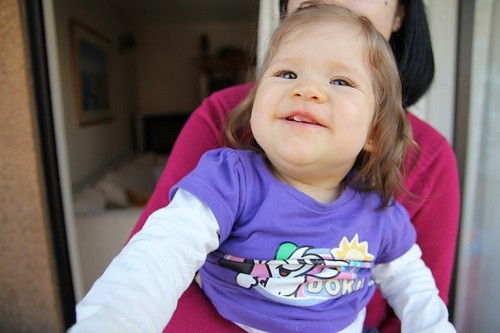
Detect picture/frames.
[67,16,114,128]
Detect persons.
[65,4,458,333]
[124,0,462,333]
